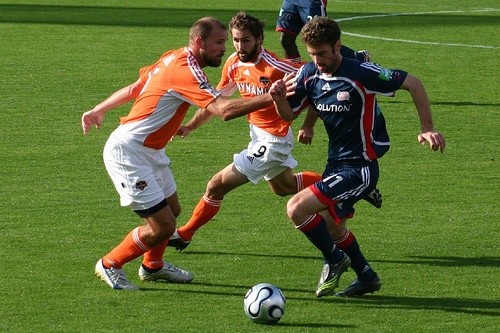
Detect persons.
[268,16,447,301]
[160,12,383,253]
[78,17,299,292]
[276,0,372,65]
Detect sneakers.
[94,258,138,290]
[334,272,382,297]
[363,186,382,209]
[315,252,351,297]
[138,259,194,283]
[169,228,190,251]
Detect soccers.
[243,283,286,324]
